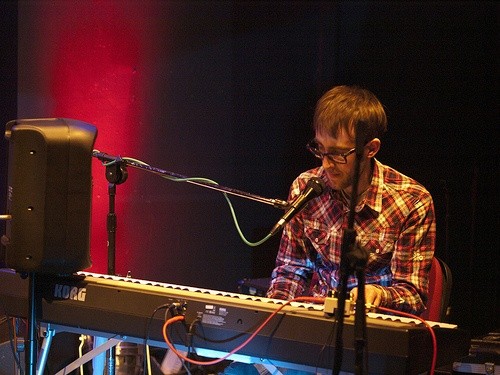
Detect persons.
[216,86,436,375]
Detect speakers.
[6,118,97,274]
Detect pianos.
[3,268,457,374]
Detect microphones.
[270,176,326,236]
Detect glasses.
[306,137,355,164]
